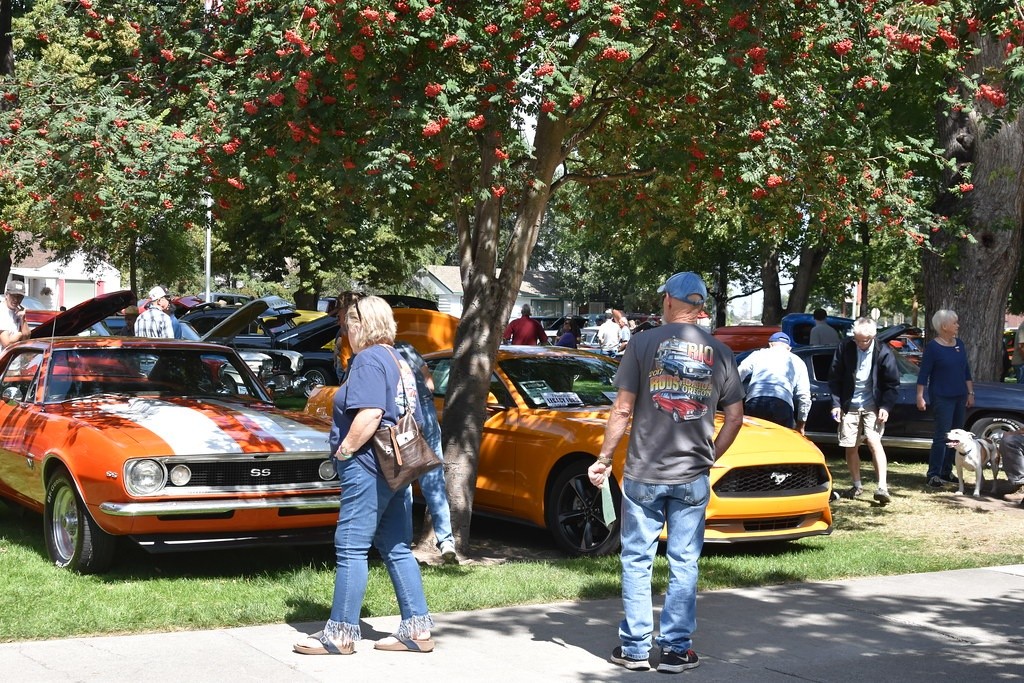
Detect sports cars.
[741,323,1024,470]
[303,344,837,561]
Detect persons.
[0,280,31,375]
[595,312,643,386]
[906,330,921,351]
[916,310,973,488]
[39,287,52,306]
[1011,321,1024,383]
[829,316,900,501]
[120,286,183,376]
[587,271,745,673]
[999,428,1024,501]
[810,309,839,345]
[294,296,437,655]
[737,332,813,435]
[554,319,581,348]
[388,343,459,564]
[329,290,354,383]
[505,304,548,345]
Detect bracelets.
[338,446,352,458]
[968,392,974,394]
[597,456,613,463]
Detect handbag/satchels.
[371,345,442,493]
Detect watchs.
[340,446,351,455]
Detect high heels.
[294,631,354,655]
[375,633,434,651]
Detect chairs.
[529,365,574,392]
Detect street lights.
[198,190,216,310]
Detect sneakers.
[441,541,460,565]
[611,646,651,669]
[940,472,964,484]
[657,649,699,673]
[926,476,944,488]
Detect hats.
[657,271,707,304]
[7,280,26,296]
[769,332,791,345]
[149,286,171,301]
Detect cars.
[0,335,341,576]
[503,312,927,373]
[0,289,441,403]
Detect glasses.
[853,338,870,344]
[353,293,368,322]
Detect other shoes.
[846,486,863,496]
[1004,485,1024,502]
[874,489,891,503]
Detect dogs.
[945,429,1000,498]
